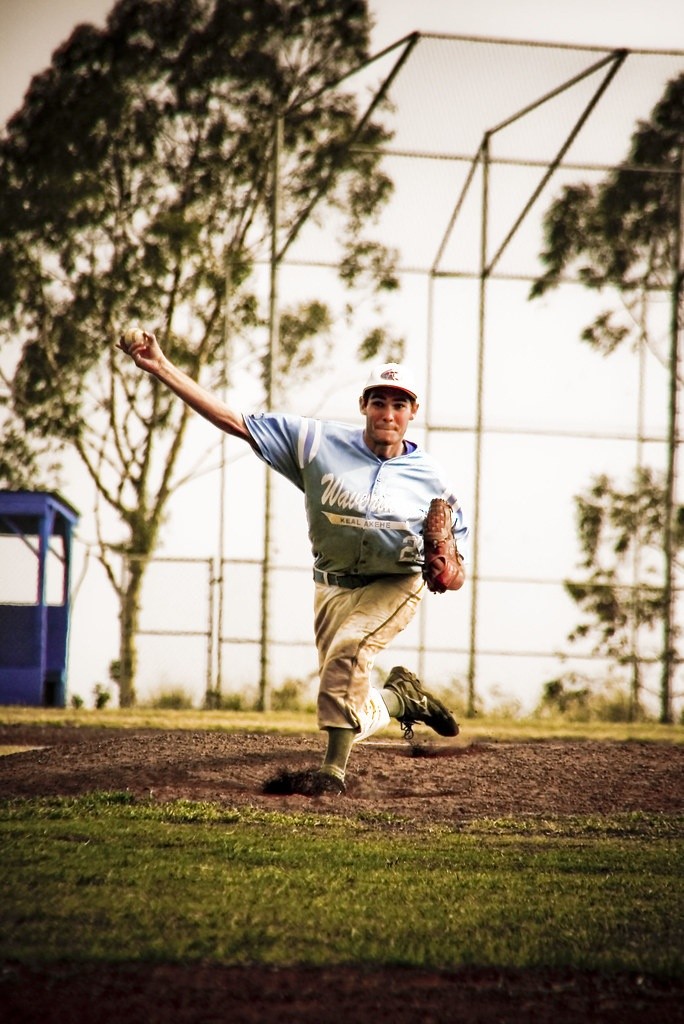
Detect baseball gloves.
[421,497,466,594]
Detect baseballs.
[123,327,147,349]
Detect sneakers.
[382,666,461,740]
[309,772,345,799]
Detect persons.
[115,332,468,795]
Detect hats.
[363,362,419,401]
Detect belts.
[314,570,398,589]
